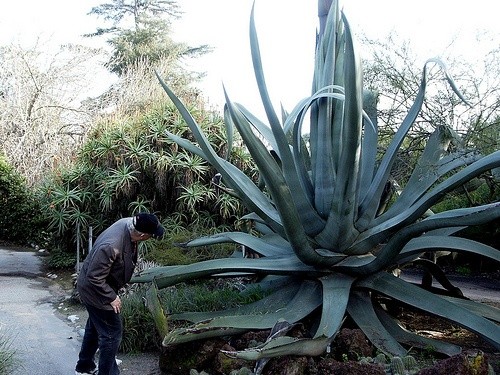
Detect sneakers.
[73,368,100,375]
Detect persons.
[75,211,166,374]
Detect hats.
[132,212,165,240]
